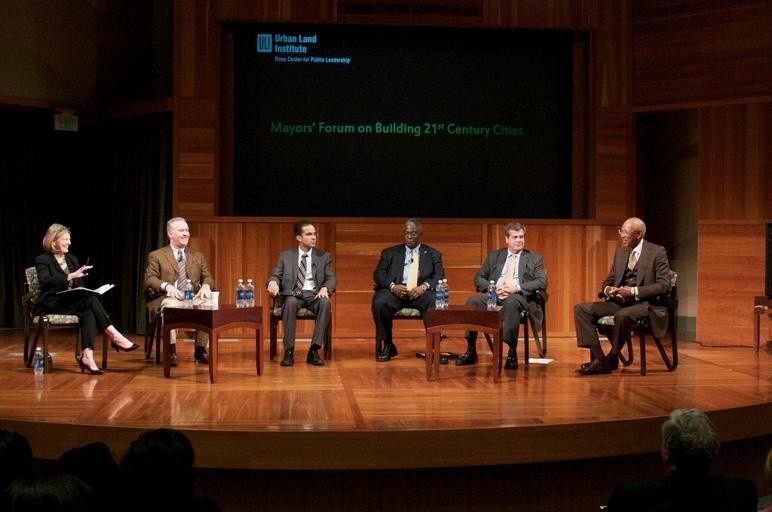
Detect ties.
[407,249,418,290]
[176,251,187,291]
[497,253,515,301]
[291,254,308,297]
[627,252,637,271]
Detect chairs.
[591,268,681,374]
[21,263,112,371]
[142,261,212,361]
[479,268,550,359]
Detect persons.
[605,407,759,510]
[760,449,772,511]
[264,220,337,367]
[139,215,217,368]
[572,215,673,377]
[0,429,225,511]
[369,217,450,365]
[454,222,548,370]
[78,373,146,424]
[29,222,140,376]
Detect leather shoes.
[505,354,518,370]
[440,357,448,364]
[194,353,209,364]
[378,343,398,361]
[575,356,620,374]
[455,352,478,366]
[307,350,323,365]
[170,352,178,366]
[280,348,294,366]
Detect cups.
[211,291,220,307]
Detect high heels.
[111,341,140,352]
[79,359,104,375]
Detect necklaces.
[58,254,67,266]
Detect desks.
[748,295,771,354]
[424,302,504,384]
[163,304,266,383]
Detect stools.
[369,288,424,358]
[269,293,333,361]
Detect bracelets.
[630,287,635,296]
[421,283,427,292]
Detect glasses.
[618,229,633,234]
[404,231,417,234]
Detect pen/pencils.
[84,257,89,274]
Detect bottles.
[183,279,194,309]
[435,278,449,309]
[236,278,246,308]
[245,278,255,308]
[34,347,45,379]
[486,280,497,309]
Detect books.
[54,283,118,298]
